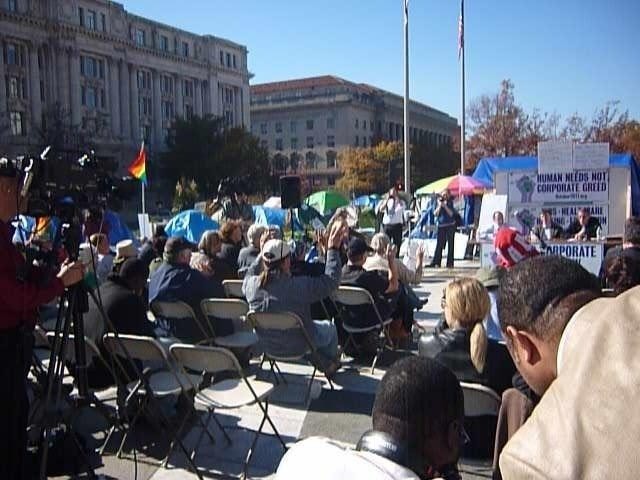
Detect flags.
[127,142,148,186]
[457,15,463,59]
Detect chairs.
[330,287,398,375]
[45,331,125,404]
[200,298,287,386]
[163,343,287,480]
[460,382,502,476]
[247,310,335,403]
[100,333,232,468]
[223,278,245,297]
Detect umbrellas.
[413,171,494,198]
[302,189,348,210]
[347,193,375,207]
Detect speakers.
[280,175,300,209]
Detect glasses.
[442,297,447,305]
[459,423,470,446]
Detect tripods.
[41,215,202,476]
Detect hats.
[262,239,291,263]
[347,238,374,253]
[165,237,196,253]
[113,239,140,264]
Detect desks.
[468,239,620,276]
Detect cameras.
[439,196,445,202]
[17,148,130,214]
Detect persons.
[426,189,463,268]
[222,190,255,226]
[486,212,504,234]
[148,236,248,369]
[242,217,342,375]
[0,157,85,470]
[109,239,139,284]
[350,232,429,313]
[88,233,114,292]
[75,257,158,391]
[495,254,639,479]
[474,266,509,343]
[26,206,57,265]
[564,207,602,241]
[141,236,167,267]
[187,230,228,286]
[379,187,407,259]
[220,219,245,269]
[606,217,639,259]
[330,234,414,354]
[532,208,564,242]
[416,277,516,470]
[617,227,640,265]
[600,251,639,301]
[237,225,269,278]
[274,356,470,480]
[72,242,101,312]
